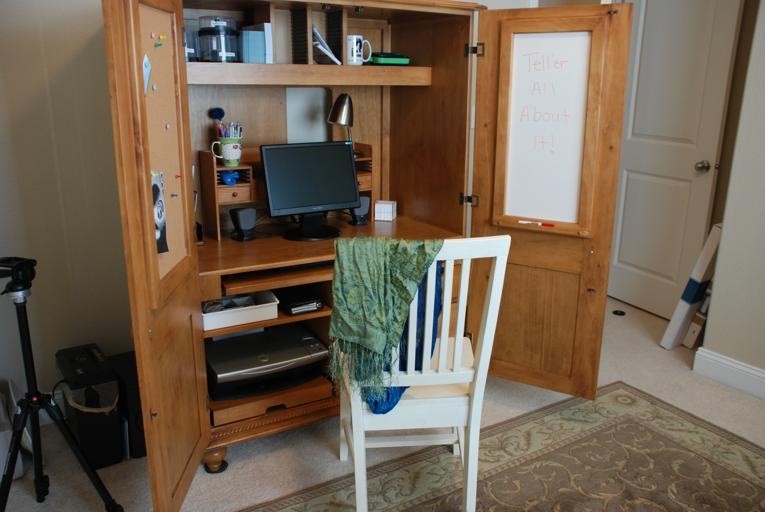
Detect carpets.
[243,377,765,512]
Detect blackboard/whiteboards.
[491,16,610,239]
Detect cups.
[210,136,243,167]
[346,35,371,66]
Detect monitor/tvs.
[259,140,361,242]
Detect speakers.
[229,207,257,241]
[349,195,370,226]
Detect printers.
[204,322,330,401]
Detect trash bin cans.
[60,380,124,470]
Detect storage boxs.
[201,290,282,332]
[238,30,266,64]
[375,199,399,223]
[0,379,39,484]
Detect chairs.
[320,229,515,512]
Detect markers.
[518,220,554,228]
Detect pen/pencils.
[208,108,242,137]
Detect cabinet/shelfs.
[91,0,638,512]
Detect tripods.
[0,258,125,512]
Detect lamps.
[323,92,362,159]
